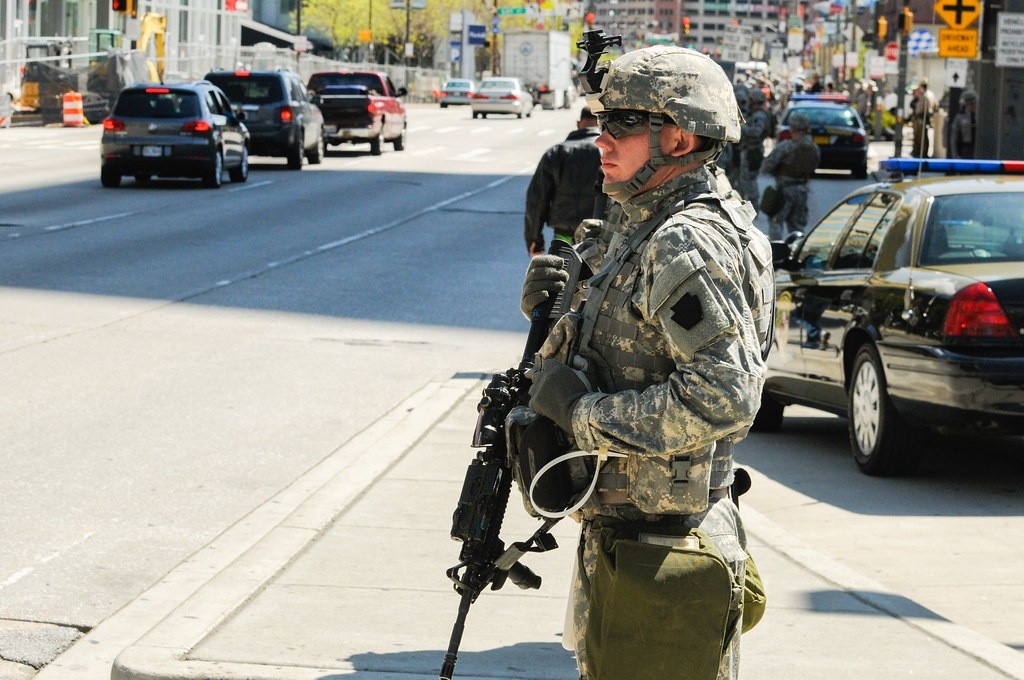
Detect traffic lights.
[111,0,133,16]
[897,6,913,35]
[876,16,888,40]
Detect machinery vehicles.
[20,11,166,111]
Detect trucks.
[501,30,575,111]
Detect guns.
[438,238,589,680]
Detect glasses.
[593,108,672,140]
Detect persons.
[789,288,829,351]
[525,106,603,258]
[808,71,877,124]
[910,78,934,160]
[761,113,820,242]
[718,70,774,214]
[519,45,777,680]
[949,89,978,158]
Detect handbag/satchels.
[760,185,786,214]
[583,512,741,680]
[503,406,607,524]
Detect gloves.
[524,358,591,435]
[520,254,571,322]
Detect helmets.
[567,30,746,143]
[788,114,811,132]
[748,90,766,104]
[960,90,976,105]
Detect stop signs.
[883,41,899,61]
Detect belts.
[599,485,733,505]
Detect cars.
[471,77,533,119]
[778,93,870,180]
[748,156,1024,479]
[100,80,251,188]
[440,79,478,108]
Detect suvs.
[204,66,323,171]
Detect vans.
[733,60,769,88]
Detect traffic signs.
[945,58,968,87]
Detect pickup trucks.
[306,71,409,156]
